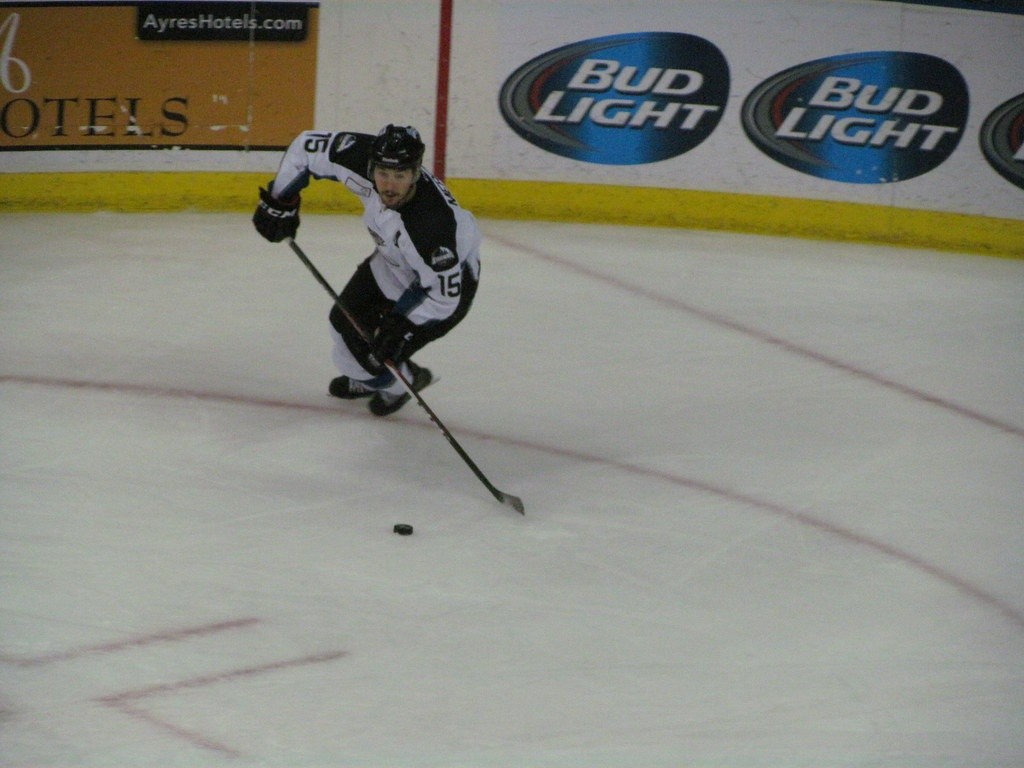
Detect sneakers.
[327,376,377,399]
[368,358,440,416]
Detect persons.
[252,123,481,416]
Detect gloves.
[352,310,420,373]
[253,180,301,243]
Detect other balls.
[393,523,413,536]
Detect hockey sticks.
[283,236,527,517]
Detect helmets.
[366,124,425,185]
